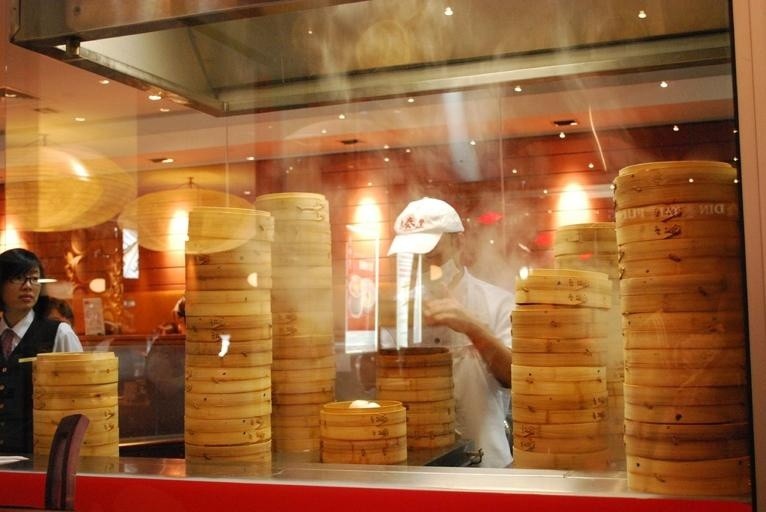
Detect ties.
[2,329,16,359]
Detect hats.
[387,197,465,257]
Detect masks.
[414,258,460,292]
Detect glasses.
[6,272,43,286]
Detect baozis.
[347,400,381,408]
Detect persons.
[34,295,74,330]
[171,295,185,333]
[334,196,517,468]
[0,247,88,454]
[145,334,186,434]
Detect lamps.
[1,131,137,233]
[116,177,256,256]
